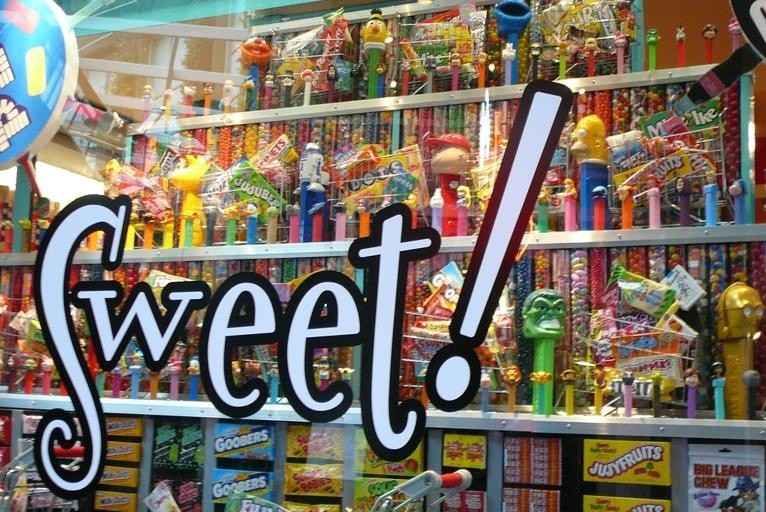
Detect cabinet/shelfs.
[1,64,765,511]
